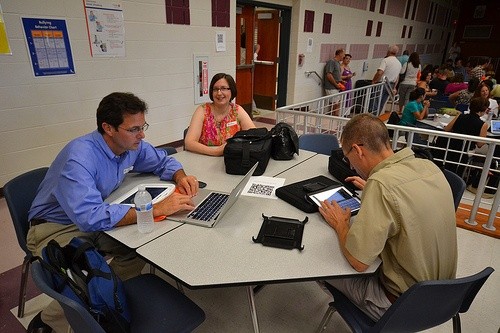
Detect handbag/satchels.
[269,122,299,161]
[329,148,363,190]
[223,127,271,176]
[399,62,408,82]
[41,238,130,328]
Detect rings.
[325,207,327,210]
[194,180,197,181]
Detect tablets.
[309,185,362,214]
[110,183,175,209]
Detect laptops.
[166,161,260,228]
[491,120,500,134]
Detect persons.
[401,87,430,127]
[397,52,421,114]
[418,57,500,155]
[340,54,355,107]
[240,32,262,115]
[372,43,402,112]
[184,73,256,156]
[323,49,345,116]
[319,113,458,320]
[26,92,199,333]
[397,50,410,65]
[449,42,461,60]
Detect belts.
[31,218,47,226]
[378,278,398,305]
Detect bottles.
[487,110,493,121]
[134,185,154,233]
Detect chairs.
[301,135,340,157]
[318,266,495,333]
[31,259,207,333]
[3,166,54,318]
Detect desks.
[418,113,449,131]
[101,149,383,333]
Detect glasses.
[213,86,230,93]
[117,122,149,134]
[341,143,364,164]
